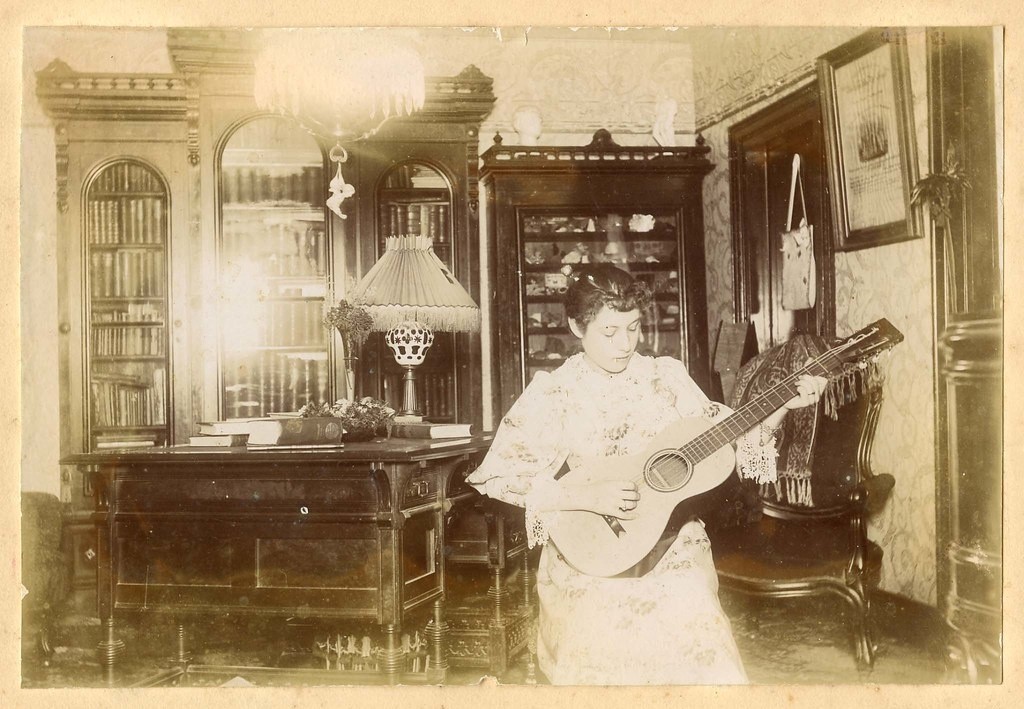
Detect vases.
[341,425,375,443]
[338,328,363,405]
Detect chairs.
[699,334,894,674]
[24,491,70,664]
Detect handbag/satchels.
[778,154,816,309]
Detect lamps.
[340,233,481,423]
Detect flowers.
[296,395,395,435]
[323,299,373,345]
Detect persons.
[464,265,827,686]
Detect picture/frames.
[818,26,922,252]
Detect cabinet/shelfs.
[480,134,716,440]
[39,26,539,680]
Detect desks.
[54,430,492,682]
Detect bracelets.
[759,420,783,446]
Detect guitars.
[532,315,906,580]
